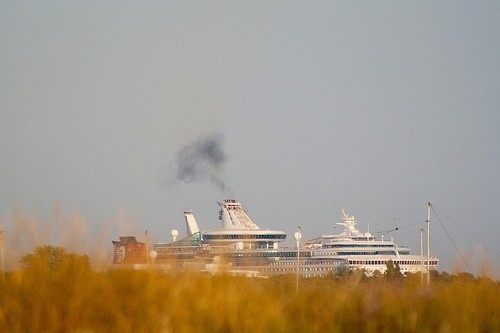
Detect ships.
[151,198,439,280]
